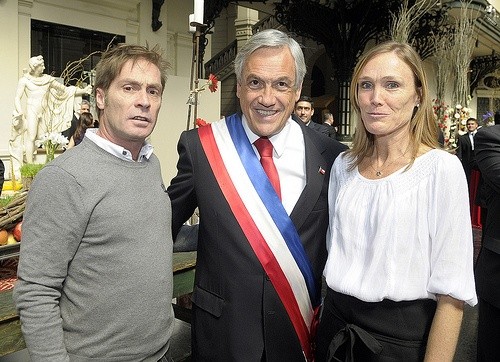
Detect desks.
[0,251,197,362]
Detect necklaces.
[370,150,411,177]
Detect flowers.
[430,99,493,149]
[34,131,69,161]
[194,74,218,126]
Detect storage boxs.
[0,242,20,280]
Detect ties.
[472,133,475,141]
[253,137,282,207]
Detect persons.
[313,41,478,362]
[164,29,350,362]
[13,43,176,362]
[61,98,100,150]
[0,158,6,198]
[293,95,341,141]
[473,109,500,362]
[7,54,94,182]
[456,118,481,169]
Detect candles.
[189,14,196,31]
[194,0,204,24]
